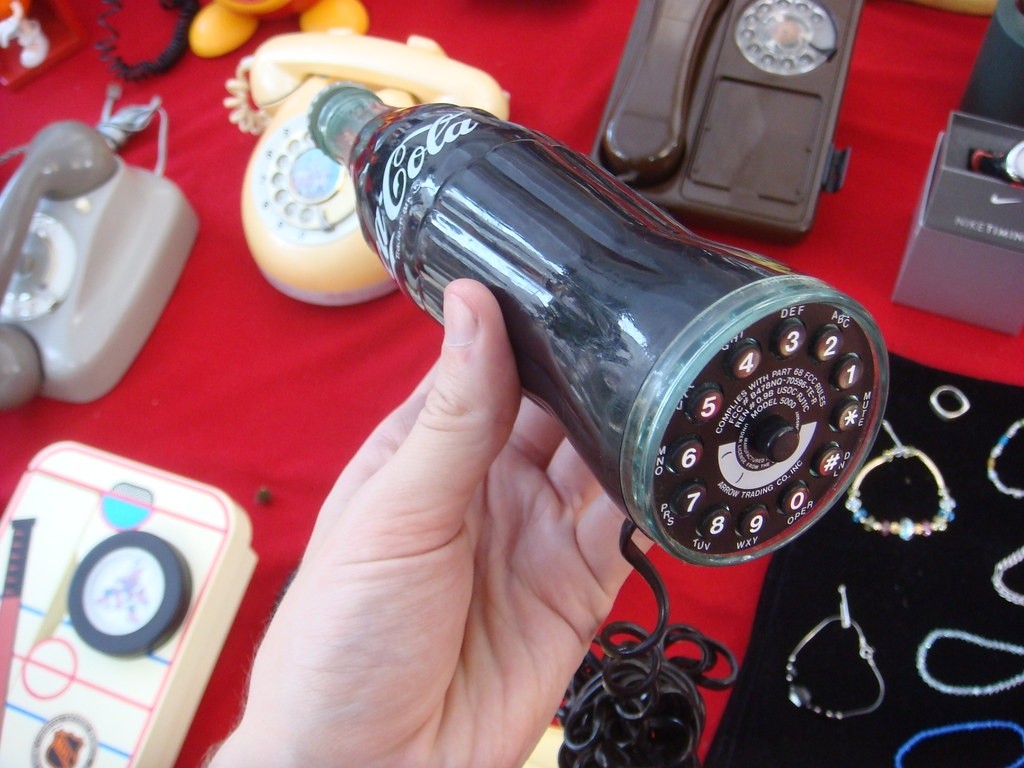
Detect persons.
[204,279,656,767]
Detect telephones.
[223,30,511,308]
[0,119,196,411]
[590,0,868,243]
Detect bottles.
[305,81,890,564]
